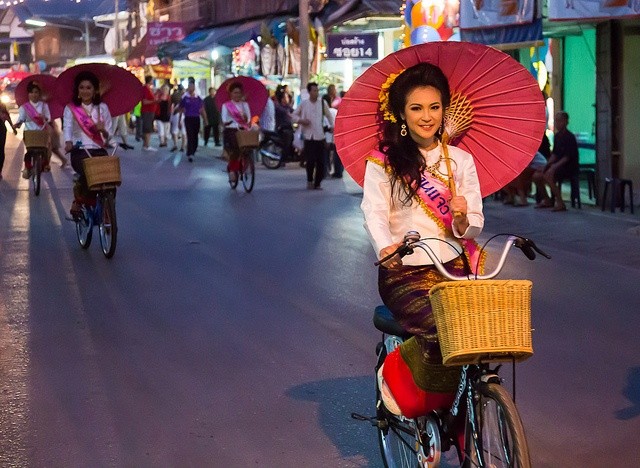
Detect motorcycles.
[259,126,299,169]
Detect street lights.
[24,16,92,57]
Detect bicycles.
[350,230,552,468]
[223,121,261,192]
[14,119,50,196]
[65,140,135,260]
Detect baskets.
[24,130,49,150]
[234,130,259,150]
[81,156,121,191]
[428,280,535,367]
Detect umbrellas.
[14,73,58,120]
[53,63,145,118]
[332,40,547,218]
[214,76,270,123]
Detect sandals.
[71,201,82,222]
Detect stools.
[578,167,597,199]
[601,176,635,214]
[551,175,581,208]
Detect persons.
[0,98,17,181]
[111,115,128,144]
[155,83,171,147]
[178,81,209,162]
[535,111,579,210]
[49,119,68,169]
[141,75,160,152]
[62,70,113,219]
[220,83,252,180]
[202,86,222,148]
[358,62,488,422]
[133,101,142,142]
[170,84,187,152]
[492,132,551,207]
[259,81,347,191]
[12,82,52,174]
[185,76,195,92]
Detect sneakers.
[551,204,565,211]
[377,363,401,415]
[308,183,313,188]
[534,201,552,207]
[504,200,515,204]
[316,186,322,189]
[142,146,157,152]
[142,146,156,151]
[514,202,527,206]
